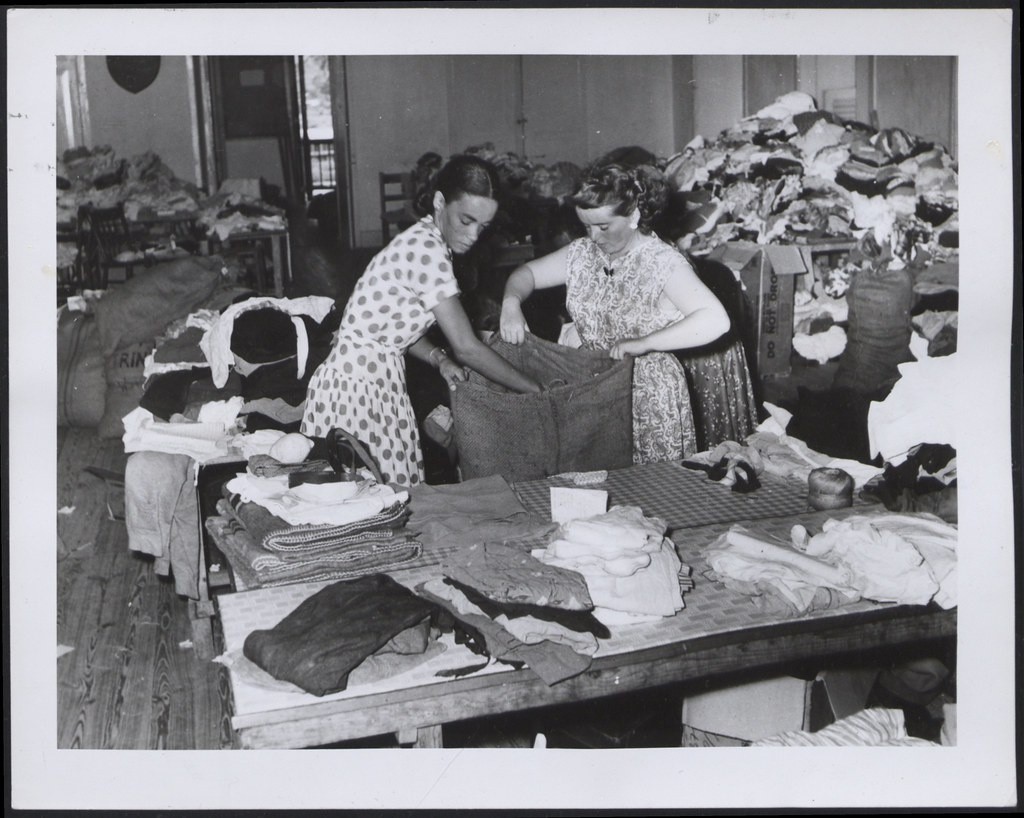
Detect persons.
[299,154,544,487]
[498,159,731,465]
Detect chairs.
[380,173,411,248]
[58,199,171,293]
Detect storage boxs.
[677,651,882,748]
[703,240,807,377]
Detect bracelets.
[429,346,447,364]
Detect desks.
[204,228,295,296]
[212,456,958,748]
